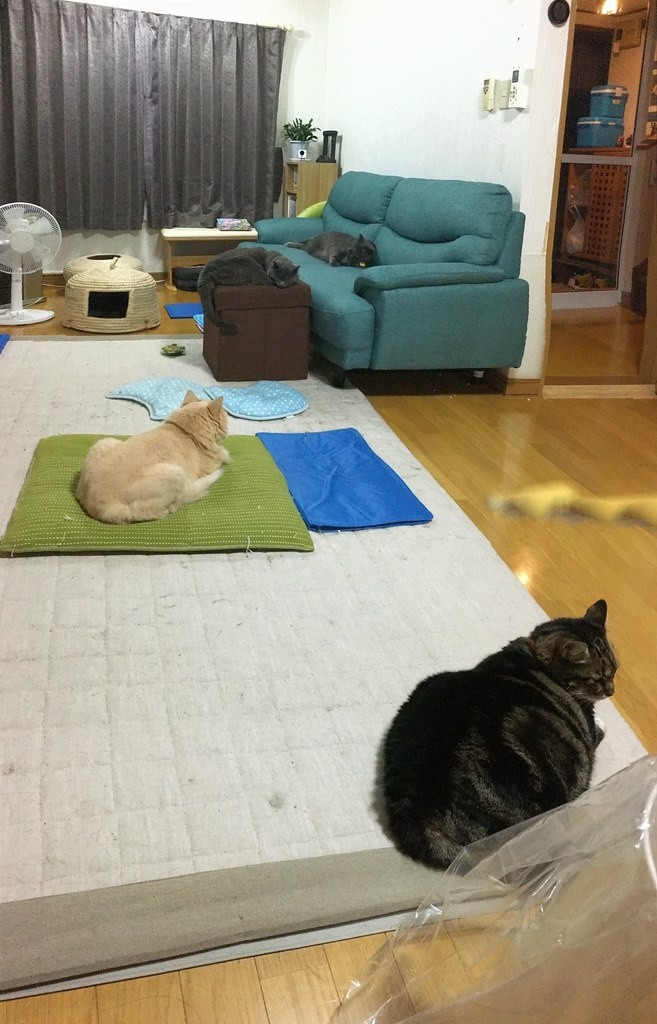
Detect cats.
[286,232,376,267]
[198,248,300,336]
[371,600,618,881]
[75,390,231,524]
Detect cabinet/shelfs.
[282,161,338,218]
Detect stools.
[202,280,310,383]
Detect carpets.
[0,334,648,1003]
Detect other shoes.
[573,273,592,289]
[576,278,608,291]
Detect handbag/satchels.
[566,205,585,253]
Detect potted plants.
[281,118,321,161]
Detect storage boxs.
[590,85,629,118]
[577,117,624,148]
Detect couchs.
[237,170,530,391]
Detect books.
[216,218,252,232]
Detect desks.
[161,227,258,292]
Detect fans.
[0,201,62,326]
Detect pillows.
[297,201,327,218]
